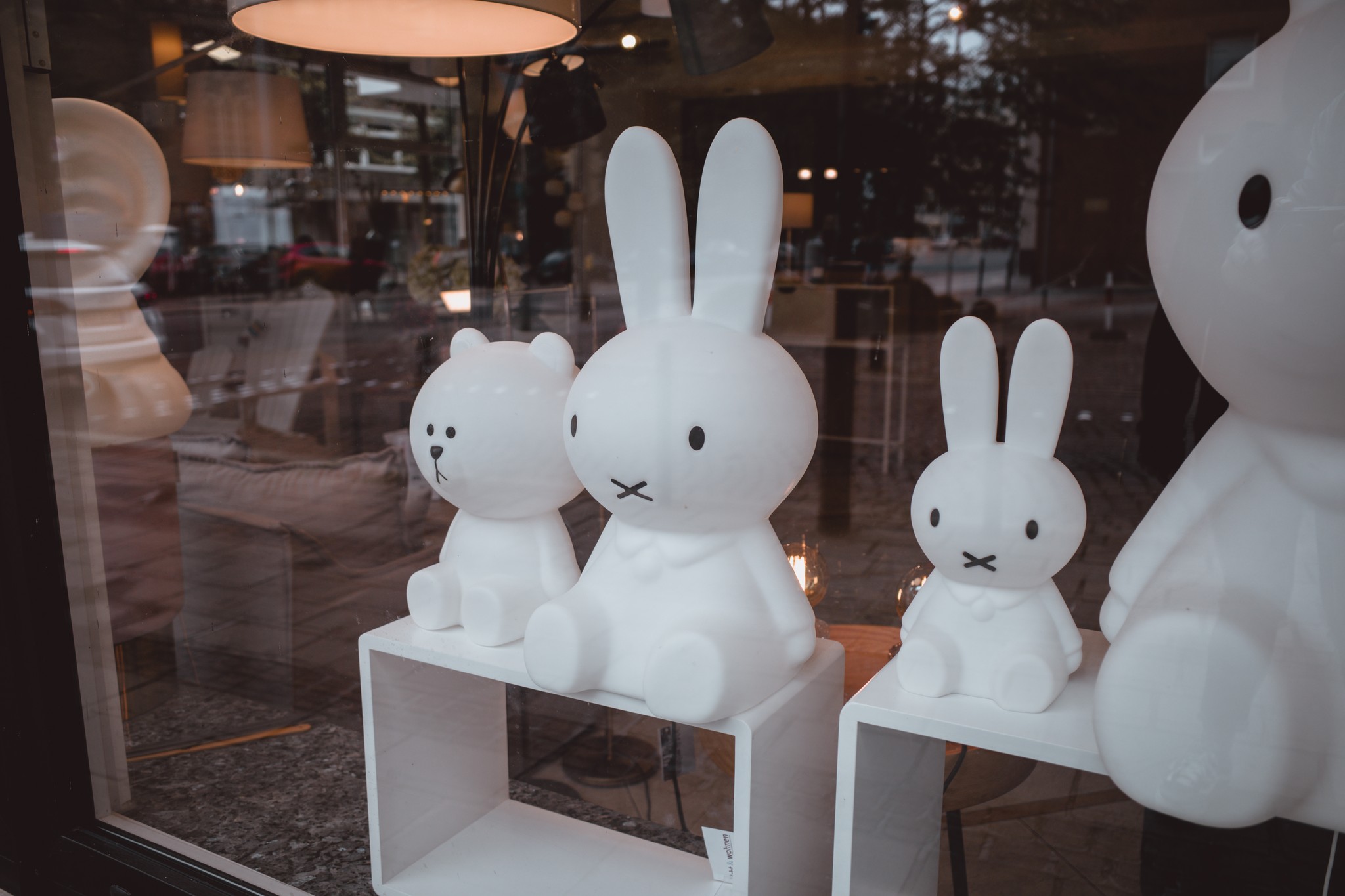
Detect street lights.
[797,168,838,283]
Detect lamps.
[776,191,814,282]
[182,69,315,169]
[224,0,586,62]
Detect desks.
[697,621,1037,896]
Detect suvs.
[16,231,175,373]
[258,238,400,295]
[140,225,279,300]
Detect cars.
[543,250,575,286]
